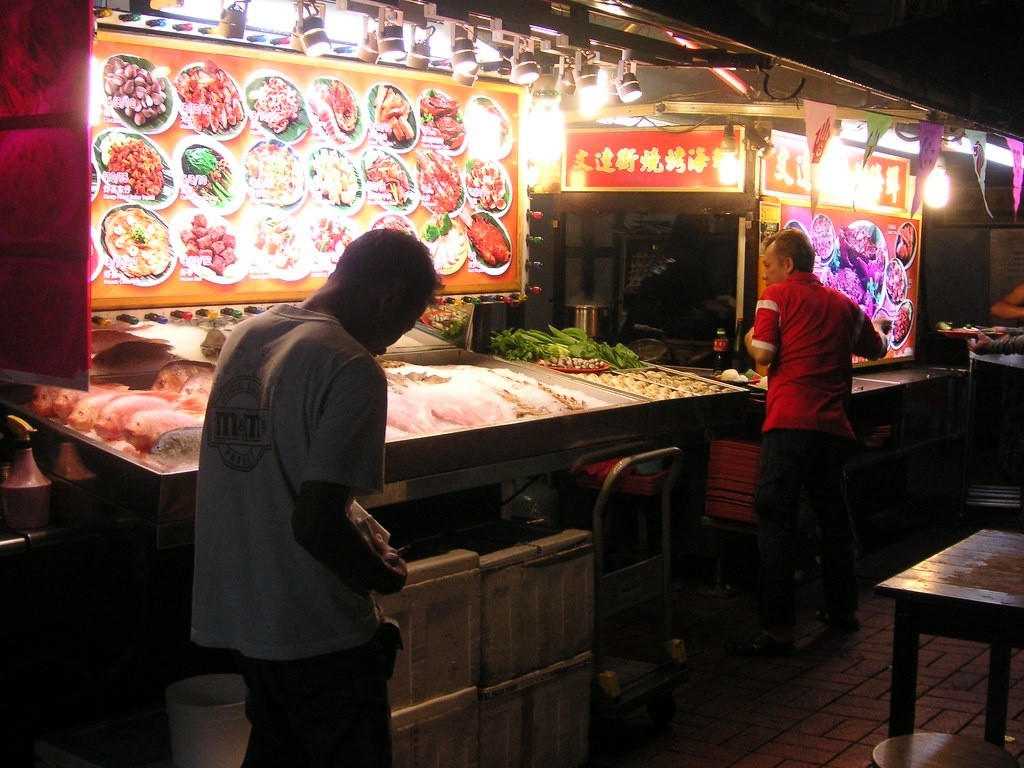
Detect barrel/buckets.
[164,674,255,768]
[566,305,609,338]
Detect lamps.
[291,0,332,58]
[356,14,379,64]
[378,6,405,61]
[668,30,756,100]
[220,0,250,39]
[612,61,641,103]
[509,36,539,85]
[448,25,477,85]
[556,55,576,96]
[571,50,599,94]
[406,24,435,71]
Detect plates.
[78,52,514,285]
[937,330,1004,338]
[783,215,920,356]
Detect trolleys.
[590,445,687,750]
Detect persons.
[963,281,1024,320]
[618,214,736,345]
[189,226,446,768]
[962,325,1024,356]
[743,227,889,644]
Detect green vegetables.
[184,147,232,203]
[485,324,643,369]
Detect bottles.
[713,328,729,373]
[732,318,745,355]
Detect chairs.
[872,733,1020,768]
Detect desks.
[874,530,1024,748]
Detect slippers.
[723,634,797,656]
[816,608,859,630]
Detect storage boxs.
[363,500,595,768]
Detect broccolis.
[426,212,452,242]
